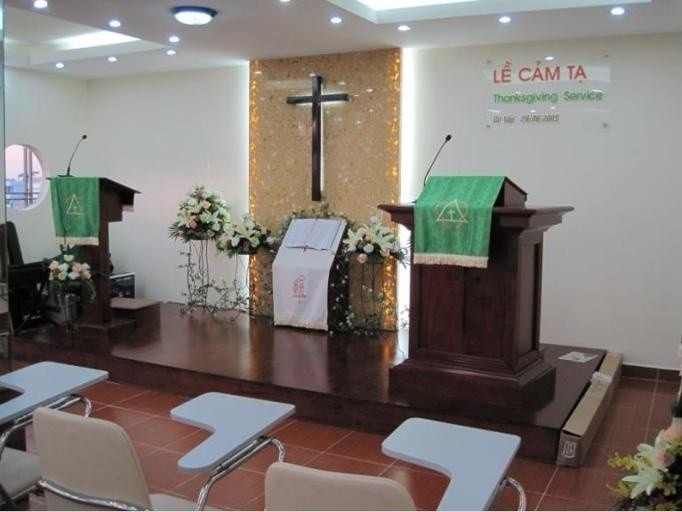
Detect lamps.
[171,5,218,27]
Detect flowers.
[169,187,276,257]
[338,215,407,263]
[49,249,97,302]
[608,371,680,510]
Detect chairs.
[264,417,527,511]
[33,391,295,511]
[0,361,108,510]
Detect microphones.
[60,130,87,176]
[424,130,452,188]
